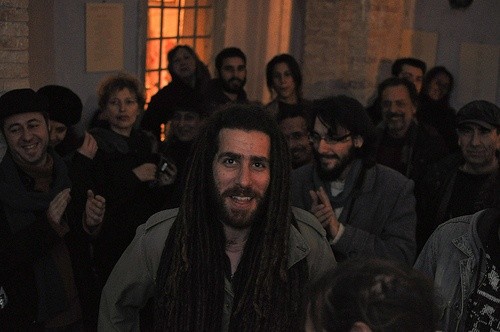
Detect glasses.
[309,131,352,144]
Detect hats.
[37,85,82,126]
[456,100,500,129]
[168,89,204,116]
[0,88,42,120]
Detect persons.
[0,53,500,332]
[99,103,337,332]
[147,44,211,128]
[192,47,247,116]
